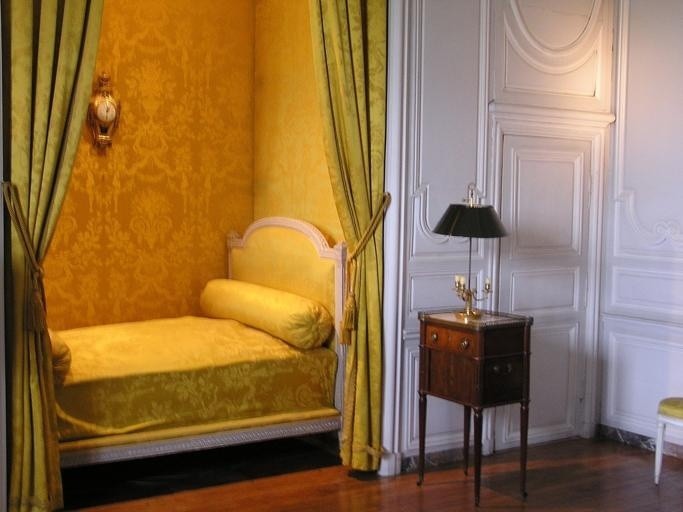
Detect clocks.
[86,68,118,151]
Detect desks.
[412,305,535,507]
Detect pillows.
[197,276,334,352]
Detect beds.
[59,215,348,470]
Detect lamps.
[430,179,510,320]
